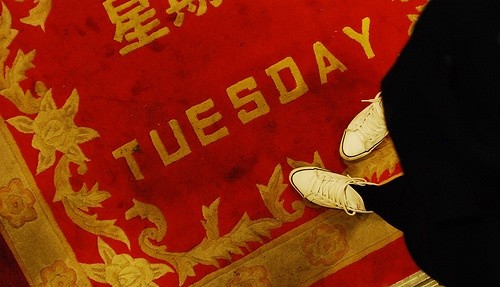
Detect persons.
[289,0,499,287]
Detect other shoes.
[289,165,375,215]
[339,98,389,161]
